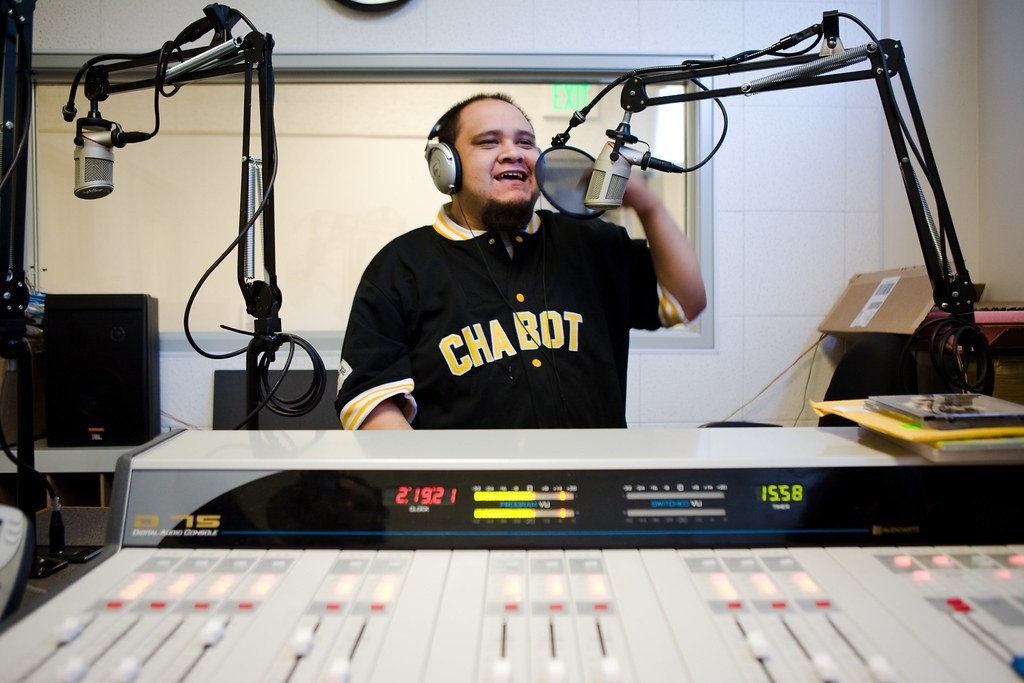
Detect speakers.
[41,293,161,448]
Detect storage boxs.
[0,356,46,450]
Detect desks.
[0,438,145,512]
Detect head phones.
[423,114,547,198]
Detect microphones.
[582,140,650,211]
[71,128,121,199]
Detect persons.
[250,475,393,552]
[333,93,707,431]
